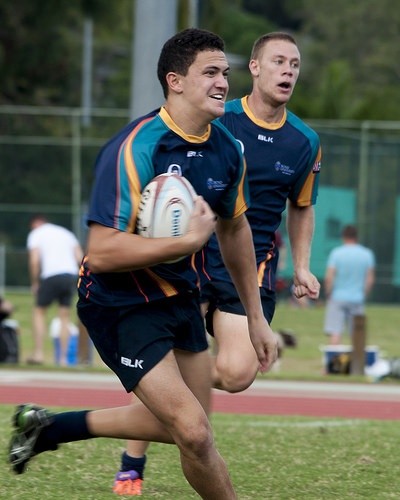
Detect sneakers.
[113,469,142,496]
[7,404,54,476]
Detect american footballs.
[136,172,197,262]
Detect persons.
[0,295,13,364]
[321,223,376,377]
[25,213,85,368]
[6,29,278,500]
[113,32,321,496]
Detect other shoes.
[27,356,43,366]
